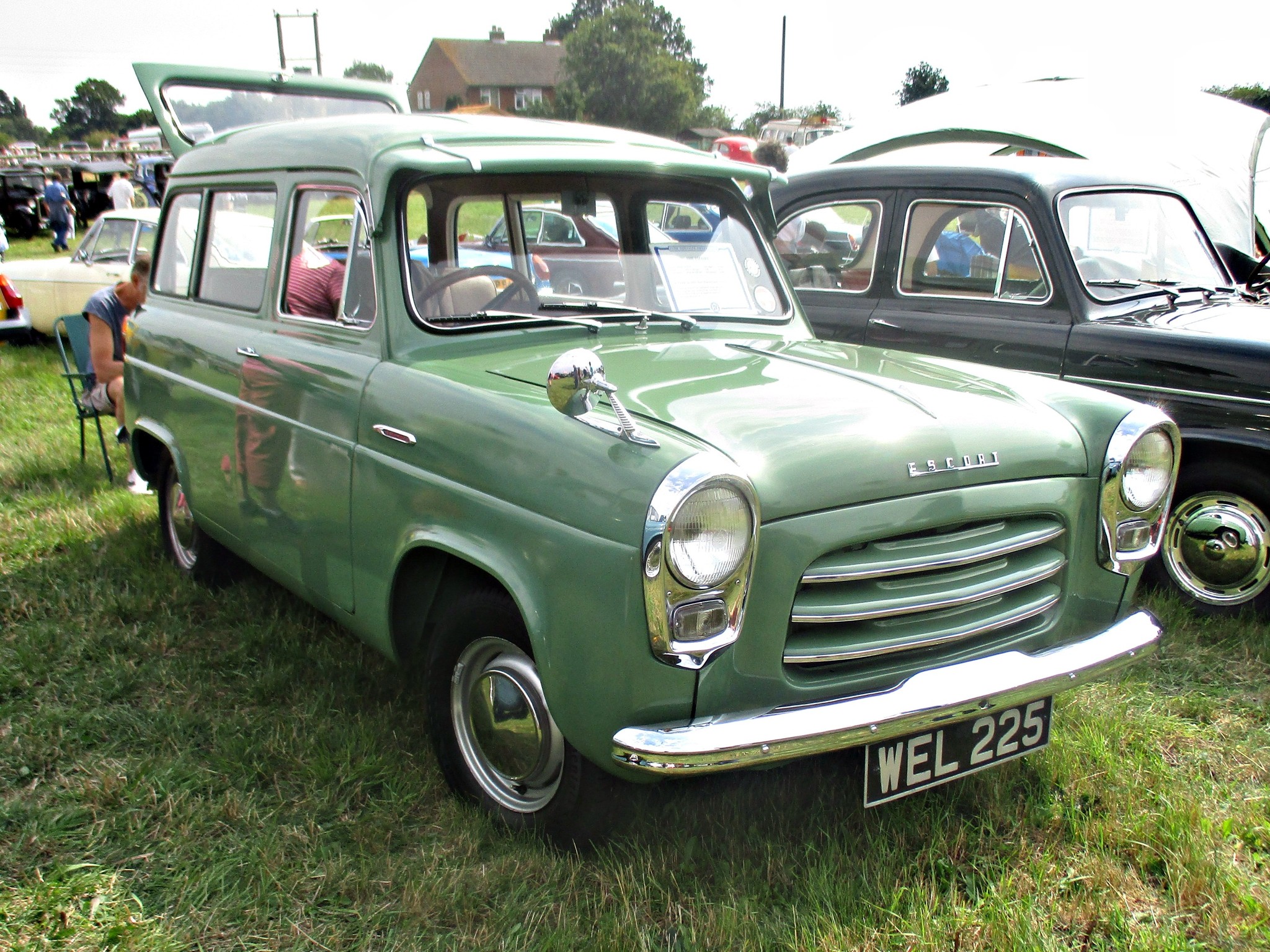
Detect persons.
[45,171,77,254]
[0,215,9,262]
[743,141,806,256]
[107,171,136,249]
[785,137,799,159]
[0,133,171,177]
[78,254,156,496]
[235,239,349,530]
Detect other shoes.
[238,500,262,517]
[261,507,290,525]
[62,247,71,250]
[127,469,153,496]
[51,242,59,252]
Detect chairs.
[53,315,134,485]
[417,269,497,315]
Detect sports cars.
[305,215,552,299]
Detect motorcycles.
[0,154,175,242]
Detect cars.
[459,71,1270,614]
[1,206,334,337]
[116,58,1184,860]
